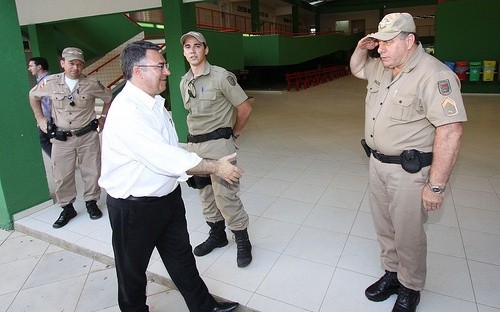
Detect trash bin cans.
[455,62,467,80]
[444,61,455,71]
[469,62,481,81]
[483,61,496,81]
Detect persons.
[179,31,252,268]
[28,57,51,157]
[29,48,113,228]
[349,13,468,312]
[97,41,244,312]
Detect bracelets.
[101,115,106,116]
[233,132,240,138]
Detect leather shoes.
[393,281,421,312]
[216,302,239,312]
[53,208,77,228]
[86,201,102,219]
[365,270,398,301]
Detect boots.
[231,227,251,267]
[194,219,228,256]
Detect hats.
[369,13,417,40]
[62,47,85,63]
[180,31,207,45]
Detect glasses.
[134,63,169,72]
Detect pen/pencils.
[77,89,79,94]
[392,89,398,97]
[201,87,203,92]
[169,119,173,127]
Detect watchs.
[426,179,445,194]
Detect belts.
[65,126,91,138]
[118,193,168,201]
[369,149,400,164]
[189,129,226,143]
